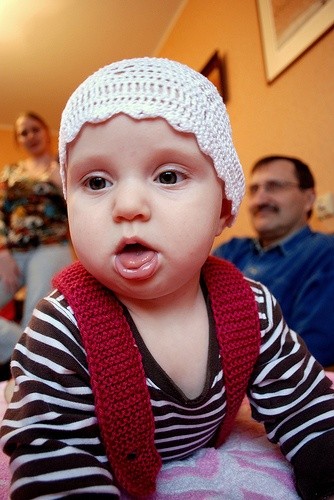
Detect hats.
[58,57,246,228]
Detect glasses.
[248,182,298,196]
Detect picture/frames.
[255,0,334,85]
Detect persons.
[210,155,334,373]
[0,112,74,382]
[0,57,334,500]
